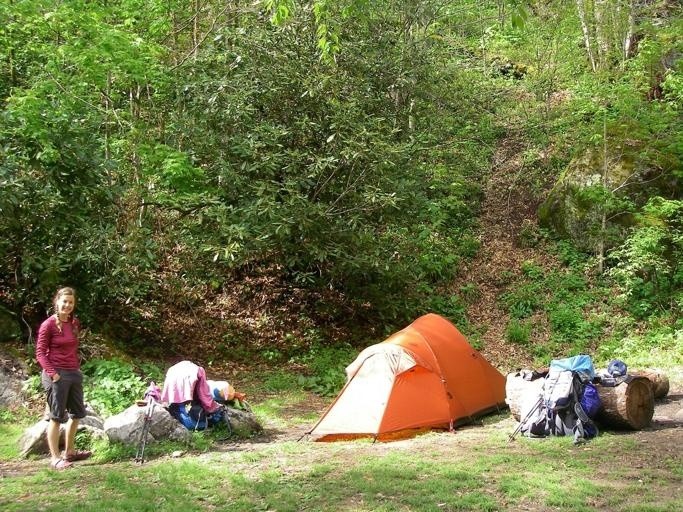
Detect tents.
[296,311,507,442]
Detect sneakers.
[50,458,73,470]
[64,450,91,462]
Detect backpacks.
[528,369,600,438]
[179,399,208,431]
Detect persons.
[34,286,92,471]
